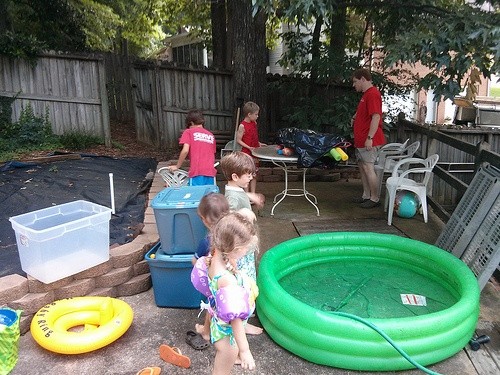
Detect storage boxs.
[146,240,217,309]
[151,185,220,256]
[8,200,112,285]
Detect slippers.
[135,367,161,375]
[158,345,191,368]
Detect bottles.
[277,148,295,155]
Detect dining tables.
[252,144,320,217]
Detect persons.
[188,190,250,365]
[235,100,267,206]
[168,108,218,186]
[202,209,258,375]
[348,68,386,208]
[213,147,266,336]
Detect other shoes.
[360,200,381,208]
[352,198,370,203]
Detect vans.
[452,96,500,128]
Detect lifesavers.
[30,296,134,354]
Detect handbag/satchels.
[275,127,349,169]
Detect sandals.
[185,331,210,349]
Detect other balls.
[395,191,421,218]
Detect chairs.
[157,162,220,188]
[373,138,440,226]
